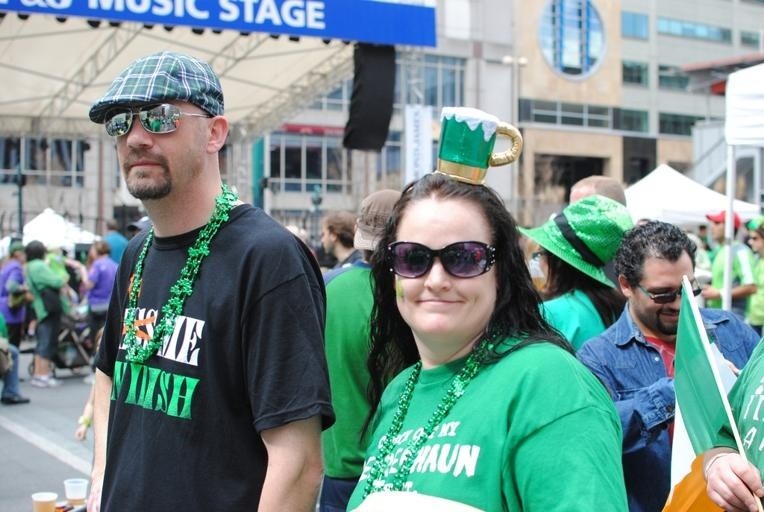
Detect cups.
[435,108,526,183]
[63,478,87,505]
[32,491,59,511]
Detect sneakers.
[30,374,64,389]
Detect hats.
[89,50,225,125]
[352,189,404,255]
[8,241,26,257]
[706,209,741,231]
[515,194,635,291]
[744,214,764,239]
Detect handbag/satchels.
[5,266,27,310]
[27,269,64,315]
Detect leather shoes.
[1,392,30,404]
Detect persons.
[1,51,764,512]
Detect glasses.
[637,275,702,305]
[385,240,500,280]
[104,102,214,138]
